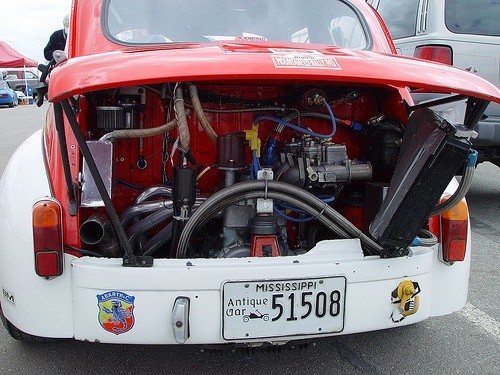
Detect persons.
[43,14,71,62]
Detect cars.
[0,1,499,351]
